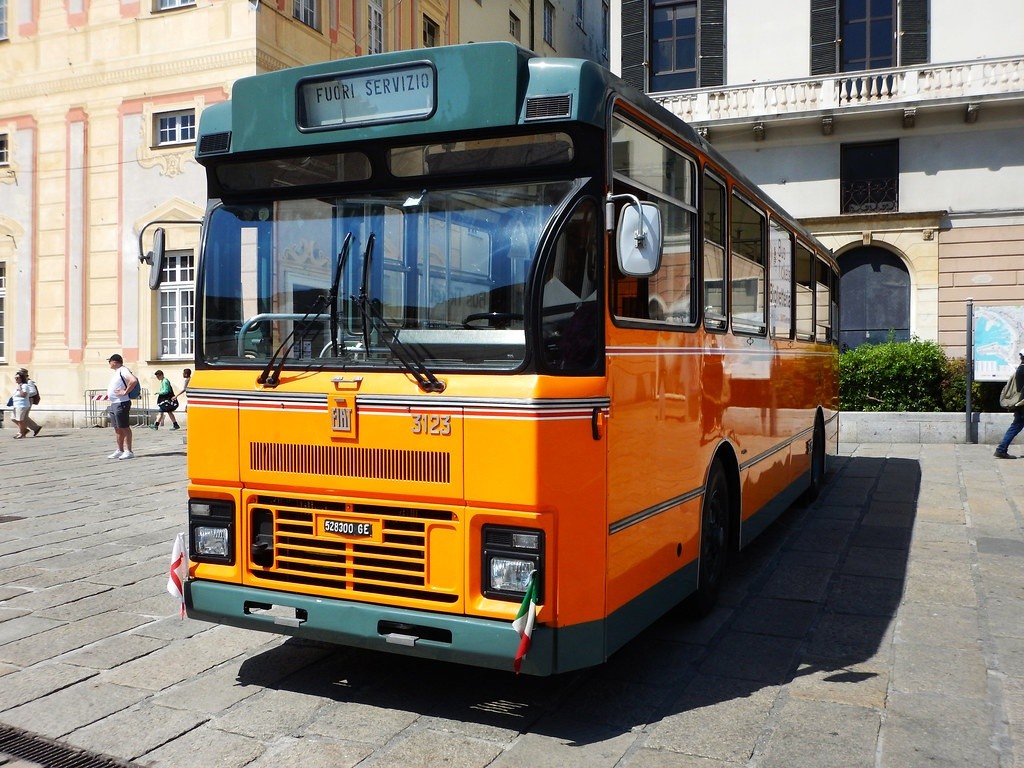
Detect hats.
[1019,348,1024,358]
[106,354,123,362]
[19,367,28,375]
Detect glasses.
[15,377,18,379]
[109,361,111,363]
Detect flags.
[512,572,536,674]
[167,535,188,620]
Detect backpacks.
[32,385,40,405]
[120,371,140,399]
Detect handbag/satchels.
[7,397,13,406]
[157,394,178,411]
[1000,371,1023,410]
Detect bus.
[138,42,840,678]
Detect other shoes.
[108,449,124,459]
[119,450,134,459]
[170,425,180,430]
[149,424,158,430]
[993,448,1017,459]
[13,434,25,439]
[34,426,42,436]
[26,429,29,434]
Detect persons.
[106,354,137,459]
[149,370,180,431]
[993,349,1024,459]
[10,368,42,438]
[172,368,191,430]
